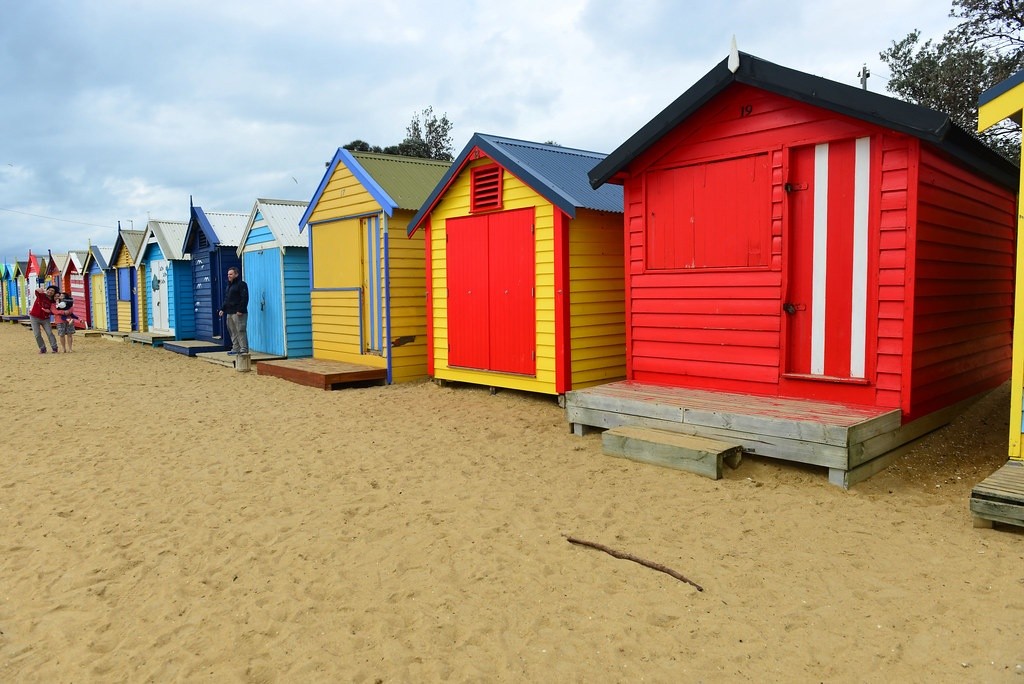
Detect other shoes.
[227,351,238,355]
[68,319,74,326]
[52,348,57,353]
[41,348,45,353]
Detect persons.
[218,268,250,356]
[56,292,83,326]
[28,285,60,355]
[50,292,75,353]
